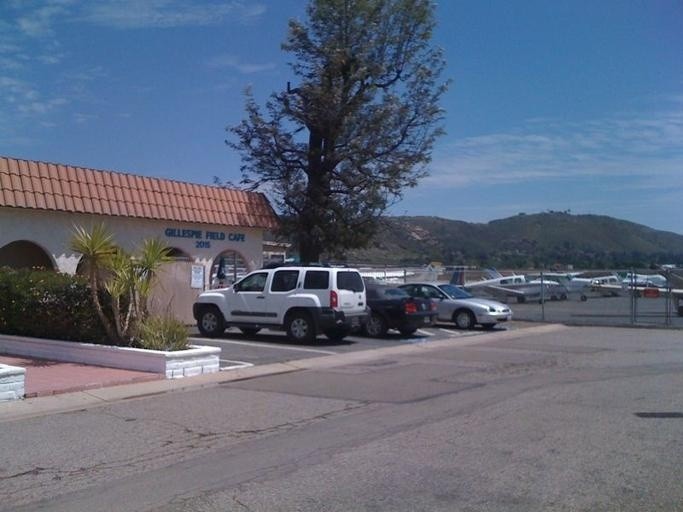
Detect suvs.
[193,264,368,344]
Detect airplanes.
[411,261,683,322]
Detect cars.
[397,281,513,330]
[348,283,439,338]
